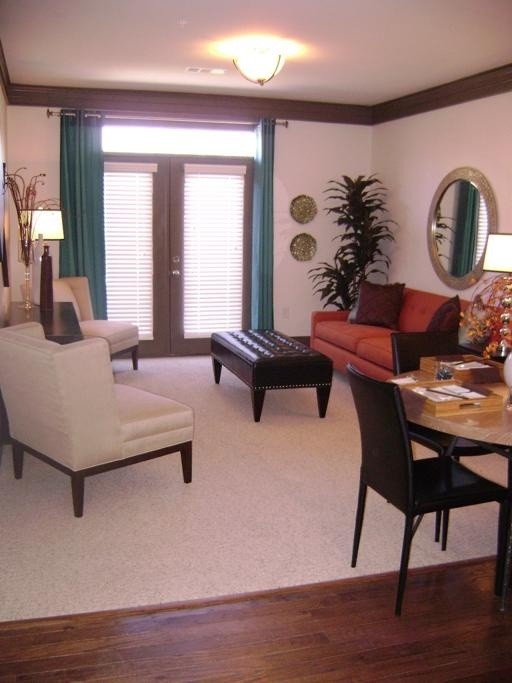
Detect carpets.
[2,354,510,621]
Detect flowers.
[2,163,63,270]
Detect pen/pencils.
[426,388,470,400]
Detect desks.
[387,365,512,615]
[449,336,512,361]
[1,300,84,446]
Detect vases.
[19,239,37,309]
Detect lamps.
[482,231,512,353]
[207,31,309,86]
[16,207,65,301]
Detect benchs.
[210,328,334,422]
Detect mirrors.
[426,165,500,291]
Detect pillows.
[425,292,461,331]
[347,278,406,330]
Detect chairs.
[389,330,512,550]
[342,360,509,616]
[1,320,193,519]
[20,276,140,370]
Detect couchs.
[309,285,512,380]
